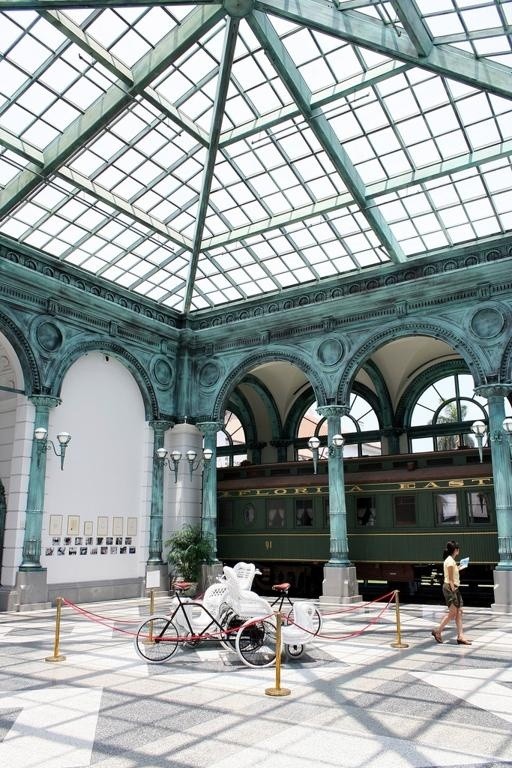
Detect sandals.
[457,637,472,645]
[432,630,443,643]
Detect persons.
[430,539,473,645]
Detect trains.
[216,446,512,601]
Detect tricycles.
[133,582,321,670]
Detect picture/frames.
[49,515,63,536]
[96,516,109,536]
[112,517,123,536]
[67,515,80,536]
[127,517,137,536]
[84,521,93,536]
